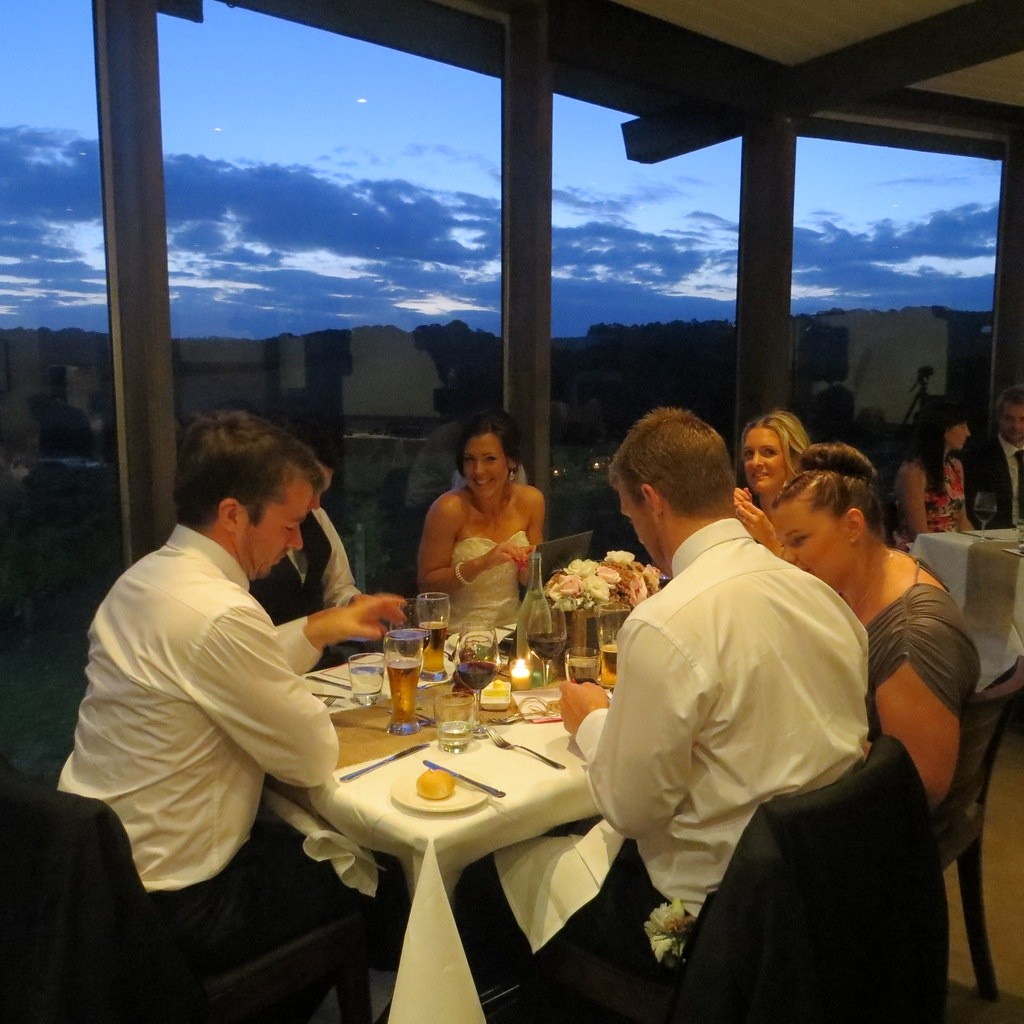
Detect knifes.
[340,743,429,783]
[423,760,506,798]
[306,675,351,692]
[311,693,345,700]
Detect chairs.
[517,733,936,1024]
[927,655,1024,1001]
[0,763,373,1024]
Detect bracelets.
[454,562,473,588]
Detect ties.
[1013,450,1023,520]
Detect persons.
[964,385,1024,530]
[458,408,869,974]
[249,427,374,672]
[417,409,546,654]
[895,396,975,553]
[732,409,811,559]
[58,409,483,1024]
[767,443,979,848]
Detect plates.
[390,768,489,813]
[479,681,511,711]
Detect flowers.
[643,897,694,970]
[543,550,664,612]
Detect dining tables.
[262,648,601,1024]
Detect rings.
[743,518,747,523]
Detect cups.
[601,602,632,689]
[509,658,535,692]
[1015,519,1024,555]
[383,629,424,736]
[433,692,475,754]
[347,652,384,707]
[564,605,602,683]
[416,592,450,682]
[390,599,420,657]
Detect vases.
[563,609,630,656]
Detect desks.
[908,528,1024,692]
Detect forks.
[486,727,566,770]
[323,697,336,707]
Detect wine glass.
[455,624,499,740]
[974,491,998,544]
[526,599,567,689]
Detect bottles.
[516,552,554,686]
